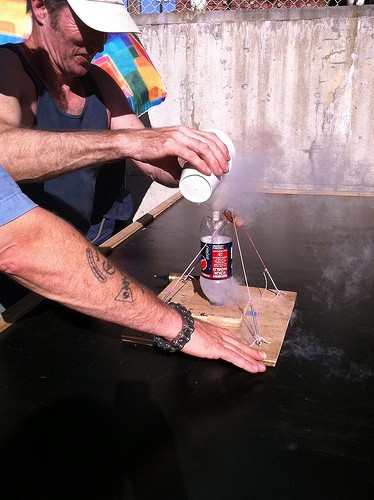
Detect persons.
[0,0,231,246]
[0,164,267,373]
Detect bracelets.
[153,301,195,353]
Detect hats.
[67,0,143,34]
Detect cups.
[179,132,236,203]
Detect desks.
[0,193,374,499]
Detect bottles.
[201,197,233,305]
